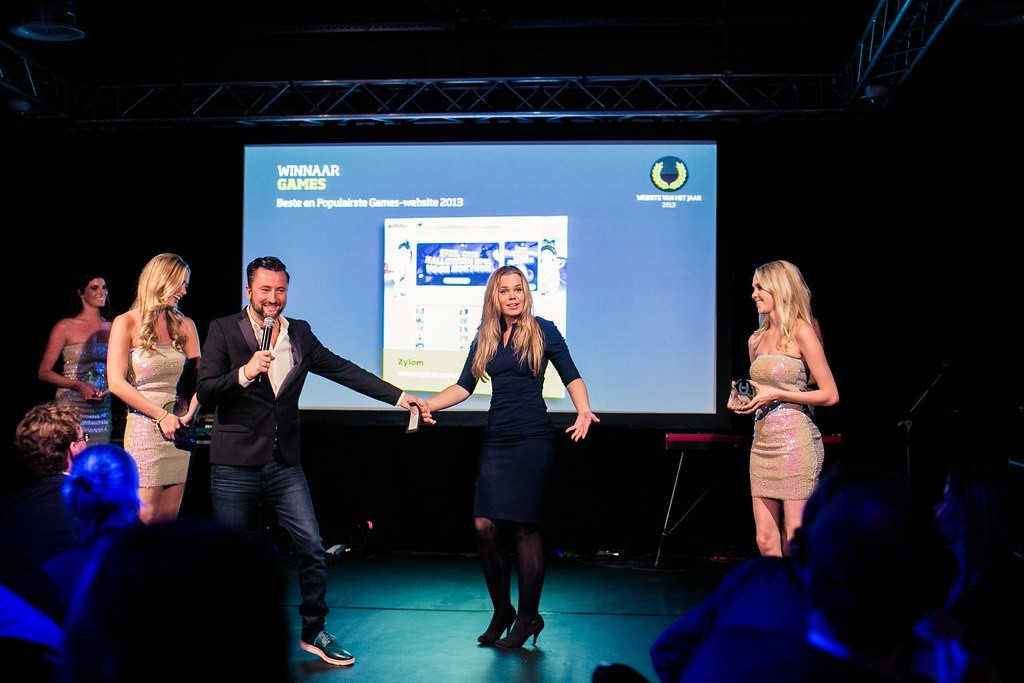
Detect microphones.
[258,316,276,383]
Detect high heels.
[496,615,544,649]
[478,609,516,643]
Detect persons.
[651,471,1024,683]
[107,252,202,521]
[36,271,114,447]
[196,255,429,665]
[0,444,294,682]
[420,266,601,647]
[728,261,839,555]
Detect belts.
[762,402,803,411]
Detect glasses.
[72,432,90,443]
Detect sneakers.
[300,623,355,666]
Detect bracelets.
[152,411,167,429]
